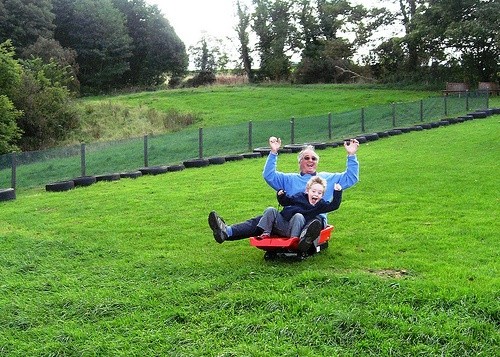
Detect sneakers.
[208,211,229,243]
[297,220,322,253]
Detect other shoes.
[257,233,271,240]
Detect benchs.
[442,81,470,97]
[474,80,500,94]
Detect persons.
[256,176,342,239]
[208,136,359,251]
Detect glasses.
[298,157,317,162]
